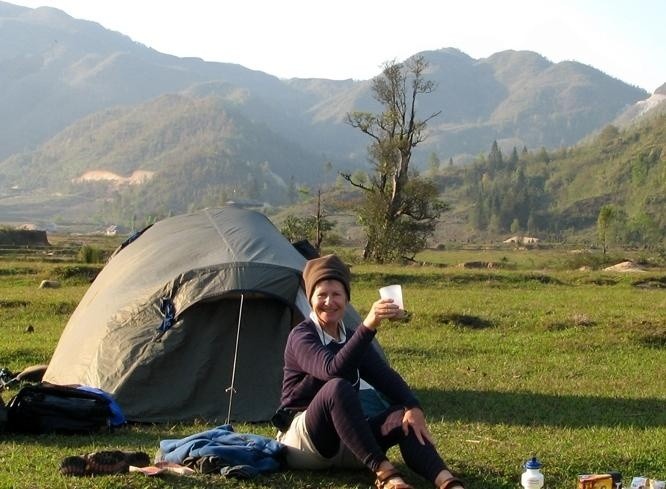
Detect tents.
[40,204,391,424]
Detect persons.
[275,252,466,489]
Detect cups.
[380,284,404,322]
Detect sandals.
[373,467,414,488]
[432,474,469,489]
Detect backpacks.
[6,381,127,435]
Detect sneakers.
[62,449,152,476]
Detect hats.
[302,253,352,300]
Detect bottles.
[521,456,545,489]
[641,478,656,489]
